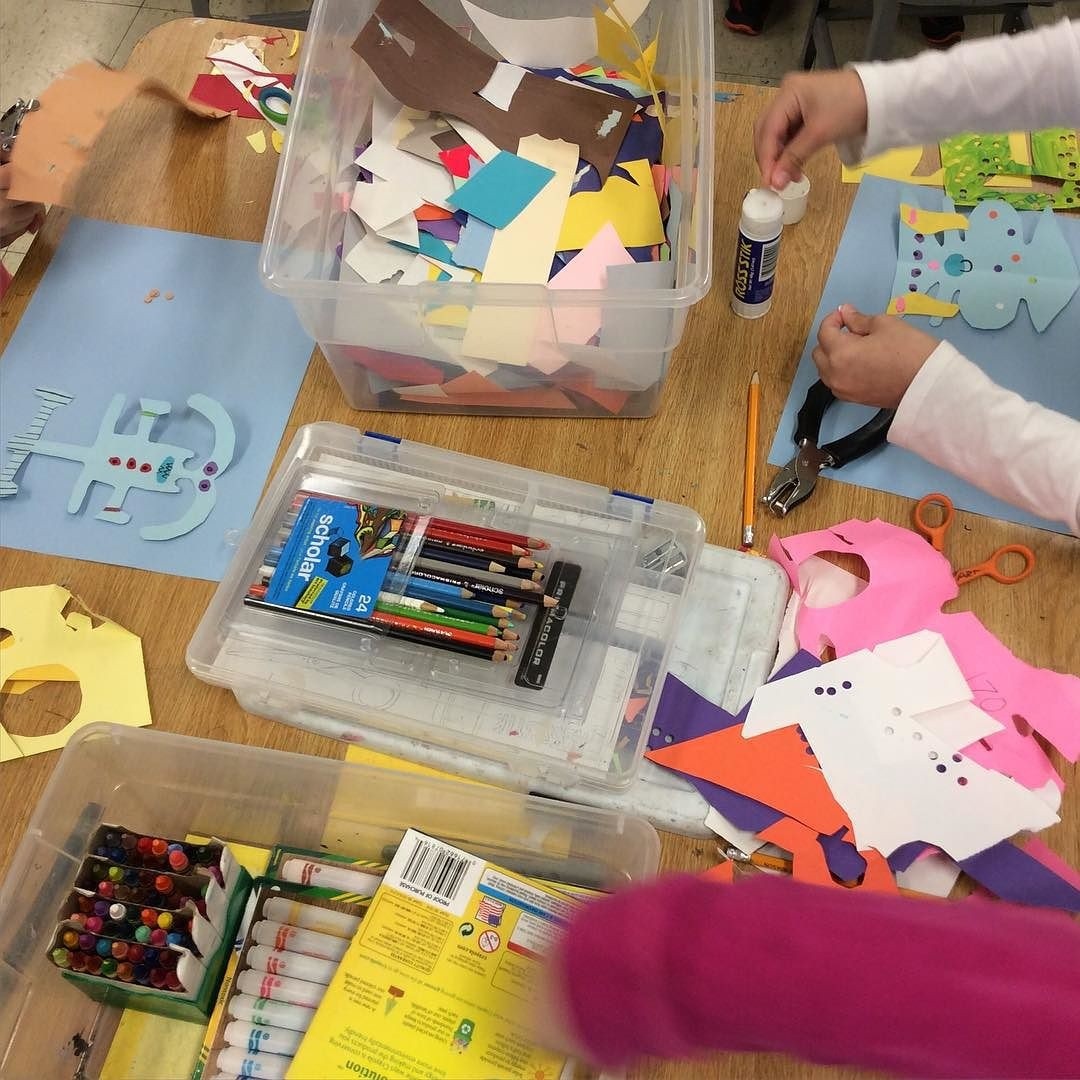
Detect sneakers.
[723,0,773,35]
[918,14,965,44]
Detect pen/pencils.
[243,488,561,664]
[724,846,943,901]
[740,370,762,548]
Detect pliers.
[758,378,897,519]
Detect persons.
[536,865,1080,1080]
[748,18,1080,541]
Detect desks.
[0,12,1080,1080]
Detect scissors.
[257,79,336,143]
[911,493,1037,590]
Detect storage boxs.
[260,0,717,418]
[0,721,663,1080]
